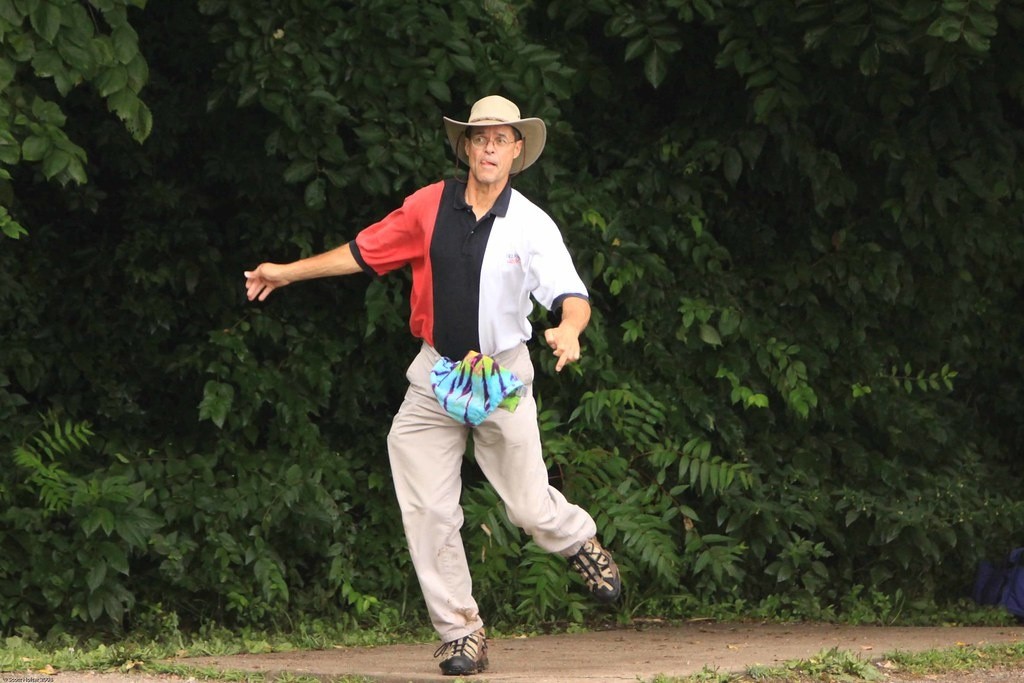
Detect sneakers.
[434,628,490,676]
[565,535,620,601]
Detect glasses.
[470,136,515,147]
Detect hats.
[442,95,547,175]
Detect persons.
[244,94,621,675]
